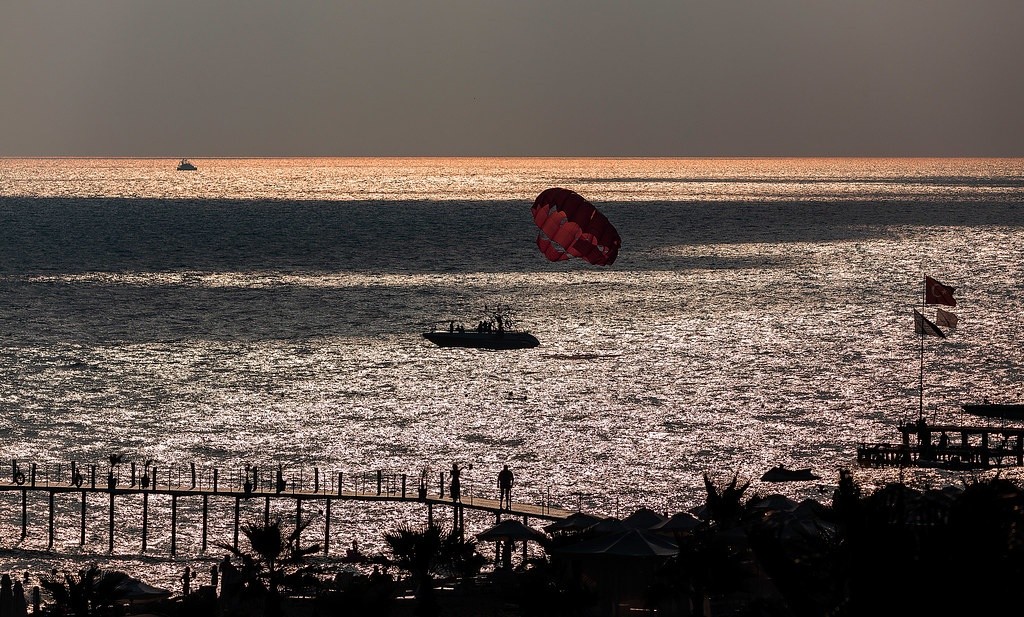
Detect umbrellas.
[476,480,1024,617]
[104,579,173,617]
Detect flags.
[925,273,957,307]
[936,308,958,328]
[914,309,947,339]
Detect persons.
[449,316,504,334]
[346,540,362,558]
[897,416,951,451]
[447,464,464,504]
[0,569,63,617]
[369,565,392,582]
[180,554,263,597]
[497,465,514,508]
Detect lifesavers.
[15,472,26,485]
[75,474,83,488]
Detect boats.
[423,326,540,349]
[177,164,197,170]
